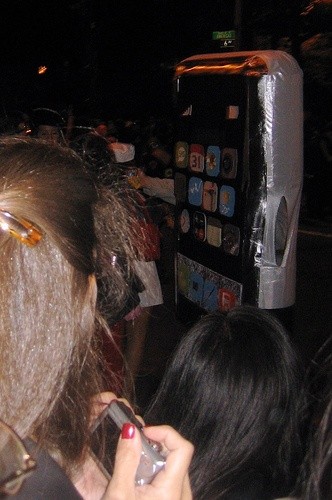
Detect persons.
[0,107,332,500]
[144,306,307,500]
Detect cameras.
[86,399,166,485]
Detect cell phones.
[171,48,303,343]
